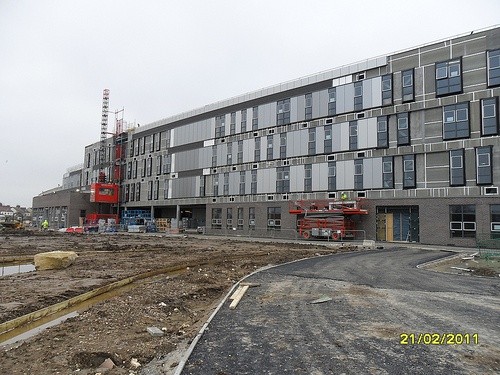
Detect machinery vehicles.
[288,199,369,241]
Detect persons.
[42,220,49,230]
